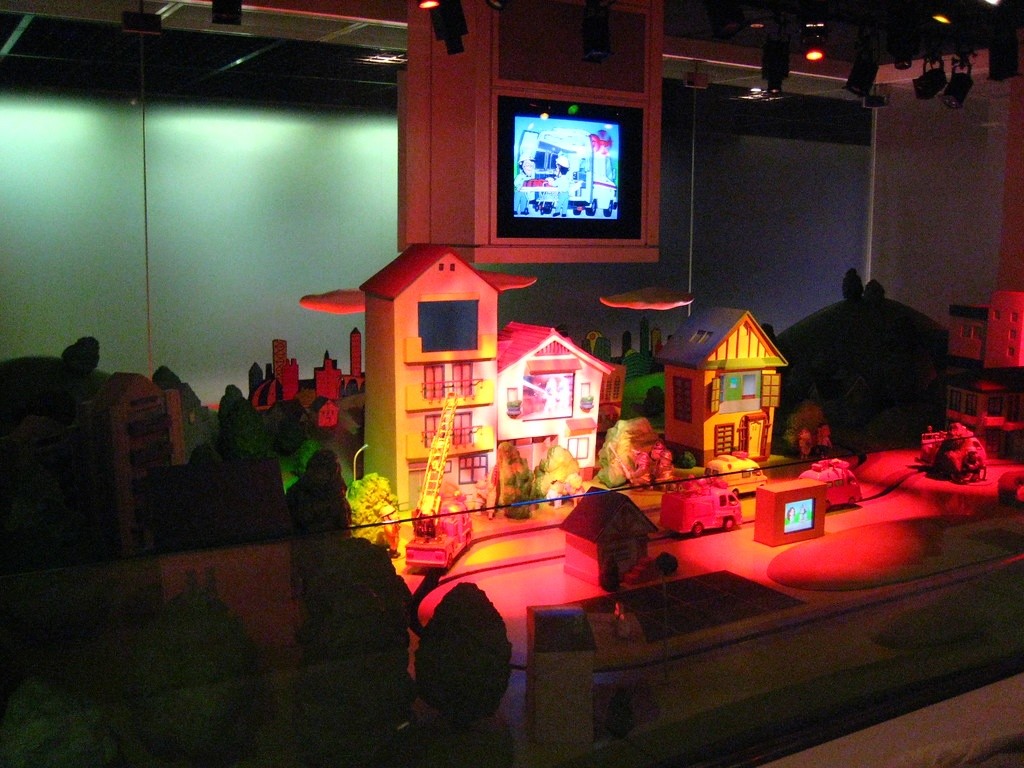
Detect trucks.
[659,484,744,536]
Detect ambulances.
[516,127,617,218]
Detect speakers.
[989,25,1020,80]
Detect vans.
[802,460,863,510]
[705,456,768,499]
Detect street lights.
[353,444,370,482]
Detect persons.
[788,507,795,527]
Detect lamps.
[417,0,510,54]
[913,43,974,108]
[885,18,923,70]
[580,0,616,64]
[840,35,880,97]
[800,12,827,63]
[760,22,791,101]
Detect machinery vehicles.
[400,393,472,571]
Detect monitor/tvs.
[490,87,649,247]
[491,0,650,99]
